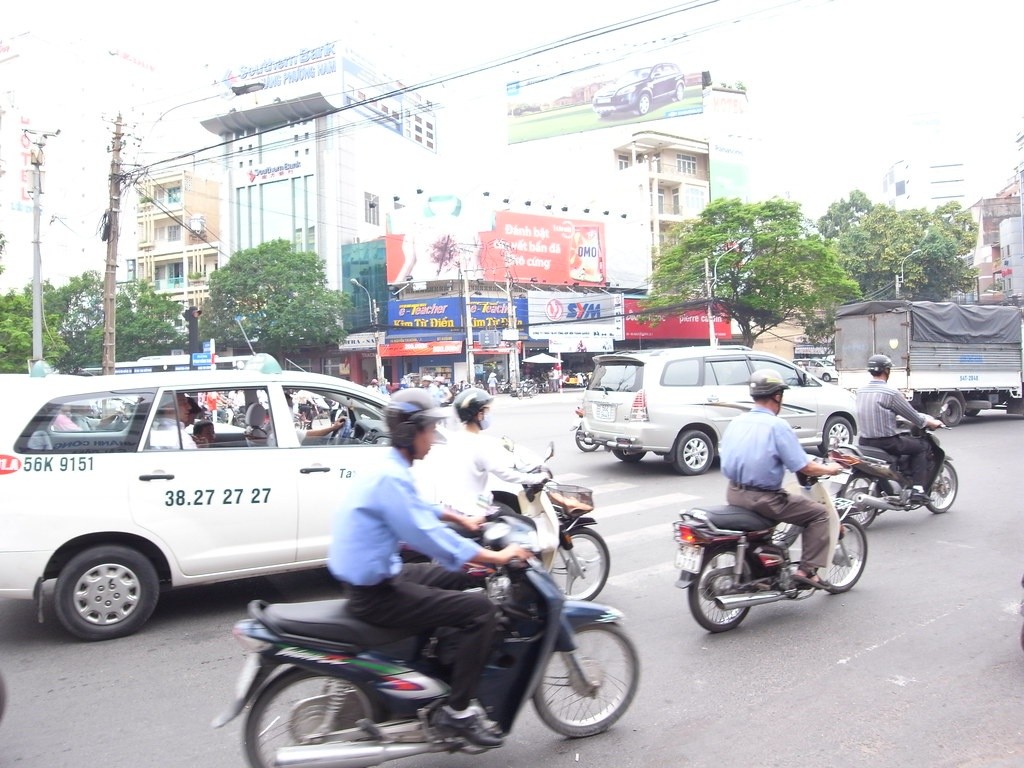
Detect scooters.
[199,398,249,429]
[400,441,613,603]
[512,380,540,399]
[572,407,603,452]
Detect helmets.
[422,375,434,382]
[452,388,494,423]
[866,354,894,372]
[371,379,378,384]
[748,369,791,397]
[385,386,447,445]
[435,376,445,383]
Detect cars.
[580,347,861,474]
[590,61,686,120]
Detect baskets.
[549,484,593,519]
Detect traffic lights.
[180,308,191,327]
[192,309,203,318]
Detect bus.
[77,351,222,376]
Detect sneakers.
[429,707,503,748]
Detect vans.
[794,358,841,384]
[0,353,488,642]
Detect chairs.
[243,403,269,447]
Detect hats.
[489,372,497,377]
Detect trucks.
[830,302,1024,426]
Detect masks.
[477,409,493,431]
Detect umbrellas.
[522,353,564,364]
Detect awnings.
[472,331,529,340]
[385,331,467,344]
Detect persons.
[436,387,560,555]
[857,355,945,503]
[267,392,346,447]
[363,363,598,407]
[150,392,216,449]
[53,400,124,432]
[719,369,844,591]
[196,389,319,429]
[324,388,532,750]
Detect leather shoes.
[910,488,935,502]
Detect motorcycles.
[211,506,642,768]
[810,404,960,530]
[670,459,871,632]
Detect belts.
[734,482,775,492]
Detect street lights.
[464,291,486,387]
[351,278,388,395]
[703,235,754,344]
[100,82,266,379]
[893,248,923,305]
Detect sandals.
[793,566,832,589]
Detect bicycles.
[293,397,332,431]
